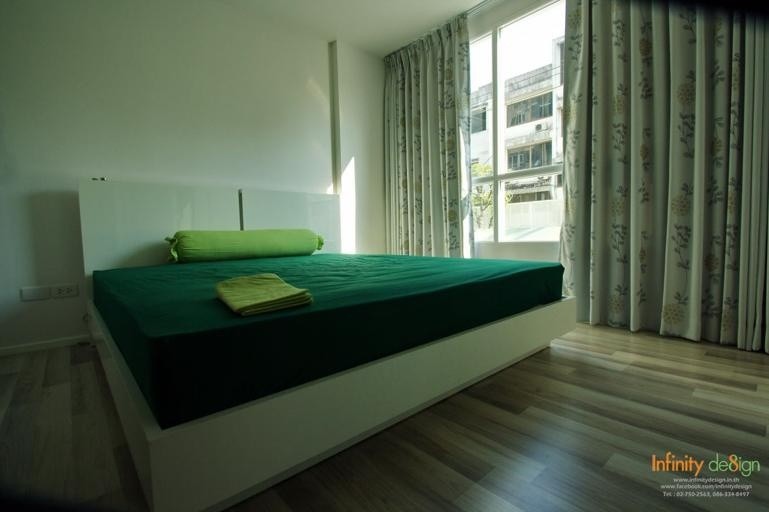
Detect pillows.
[164,228,324,262]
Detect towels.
[215,273,312,316]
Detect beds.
[77,177,578,512]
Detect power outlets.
[51,284,78,298]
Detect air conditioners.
[534,122,548,132]
